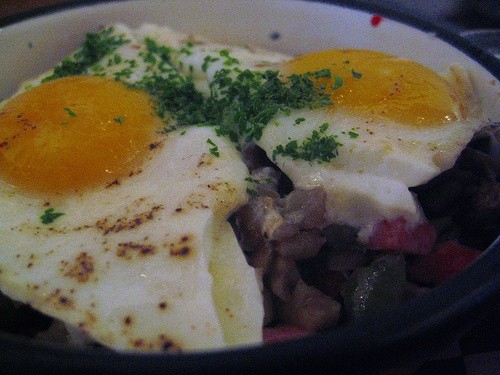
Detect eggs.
[142,22,488,189]
[0,23,268,353]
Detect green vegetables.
[22,25,366,226]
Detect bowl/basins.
[0,0,500,375]
